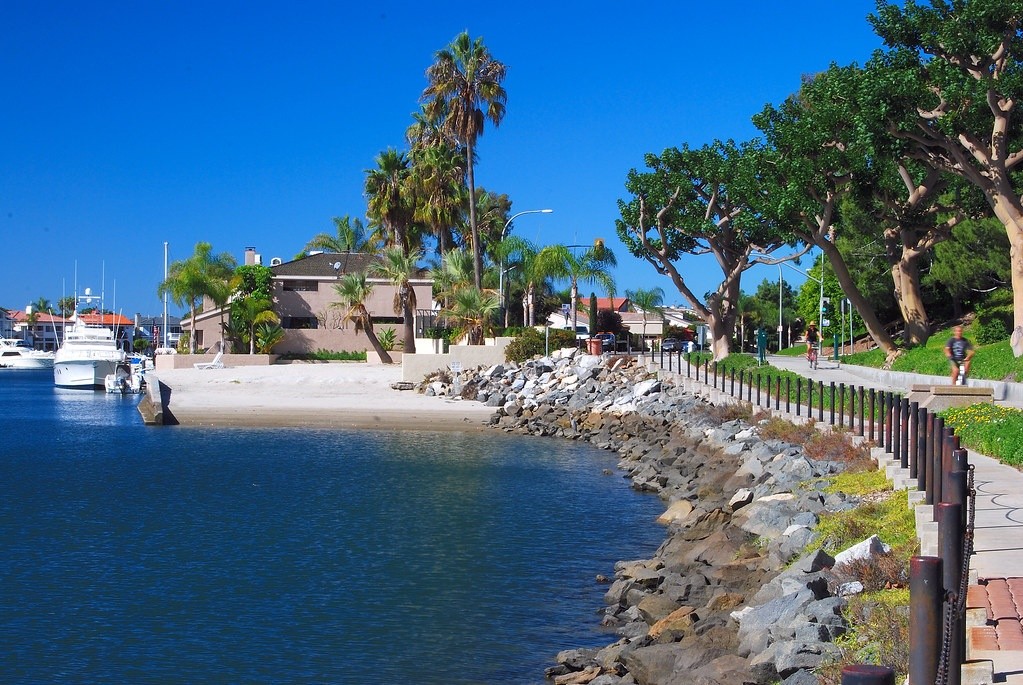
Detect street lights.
[751,251,783,352]
[500,209,554,305]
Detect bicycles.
[806,340,823,370]
[950,357,970,385]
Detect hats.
[809,321,817,326]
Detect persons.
[945,326,974,385]
[805,320,824,365]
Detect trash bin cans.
[585,338,602,355]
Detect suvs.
[662,338,683,352]
[593,333,615,351]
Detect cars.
[679,341,697,352]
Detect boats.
[104,362,143,394]
[54,289,128,391]
[0,339,55,368]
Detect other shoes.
[806,354,810,360]
